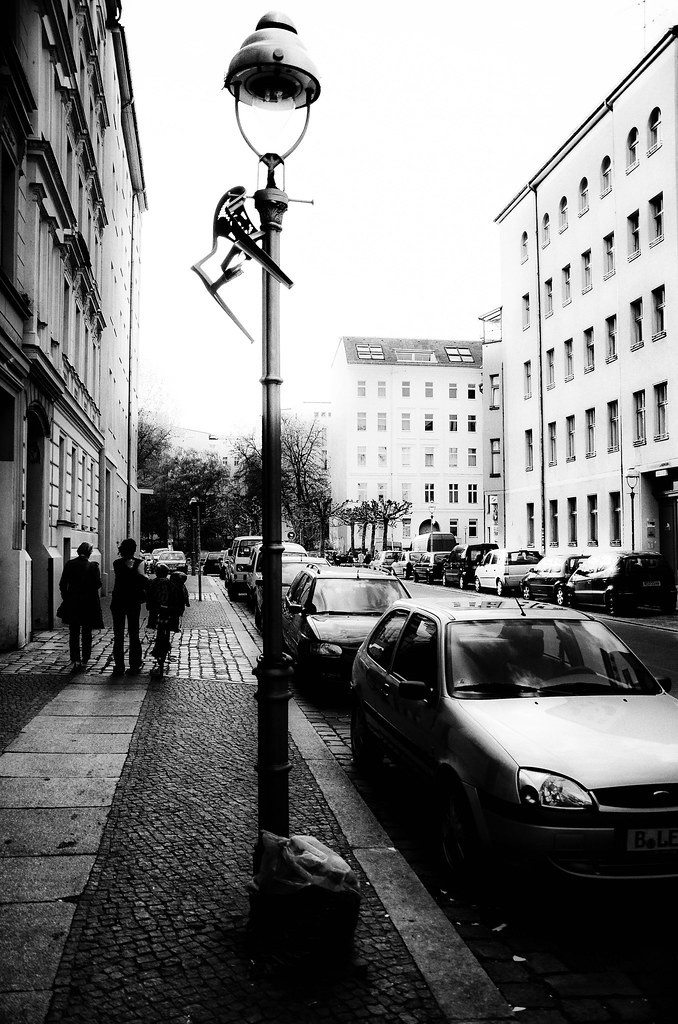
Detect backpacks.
[112,559,149,610]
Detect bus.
[411,532,457,552]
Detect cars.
[389,551,428,580]
[309,547,374,566]
[563,550,677,616]
[280,562,432,695]
[198,551,232,579]
[369,551,404,573]
[154,550,192,575]
[140,548,169,574]
[412,552,452,584]
[473,548,545,597]
[518,554,594,608]
[349,595,678,914]
[254,555,332,631]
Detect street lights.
[428,501,437,552]
[220,11,323,948]
[625,467,640,552]
[189,497,206,603]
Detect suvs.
[224,536,266,599]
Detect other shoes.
[127,665,141,676]
[75,661,87,667]
[157,636,171,649]
[113,666,125,676]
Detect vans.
[440,543,499,589]
[247,542,309,608]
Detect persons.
[333,549,378,568]
[57,542,105,667]
[110,538,148,676]
[170,571,190,613]
[146,564,182,633]
